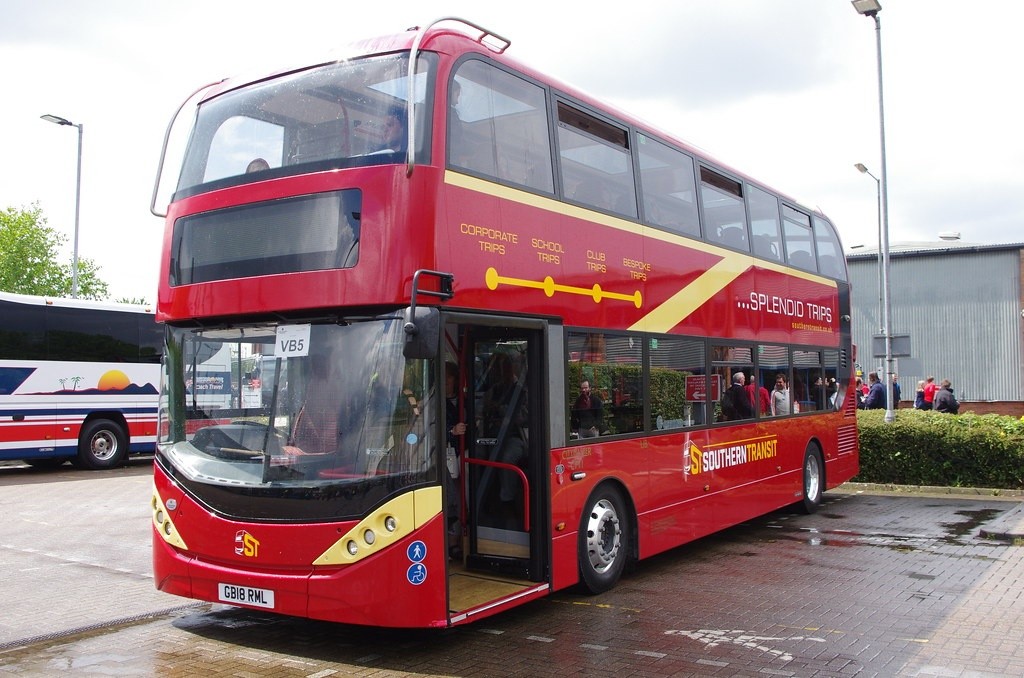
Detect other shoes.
[449,545,463,560]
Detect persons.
[448,79,463,167]
[262,342,406,475]
[446,361,477,563]
[722,372,800,421]
[363,104,408,154]
[230,385,239,409]
[473,349,531,519]
[570,379,603,439]
[933,380,960,414]
[855,371,901,410]
[244,158,269,173]
[915,376,935,411]
[813,376,847,409]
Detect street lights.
[851,0,895,424]
[38,111,83,298]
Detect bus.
[149,18,861,627]
[232,355,288,415]
[0,292,232,469]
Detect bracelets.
[450,427,453,435]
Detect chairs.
[265,355,346,480]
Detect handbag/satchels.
[446,425,459,478]
